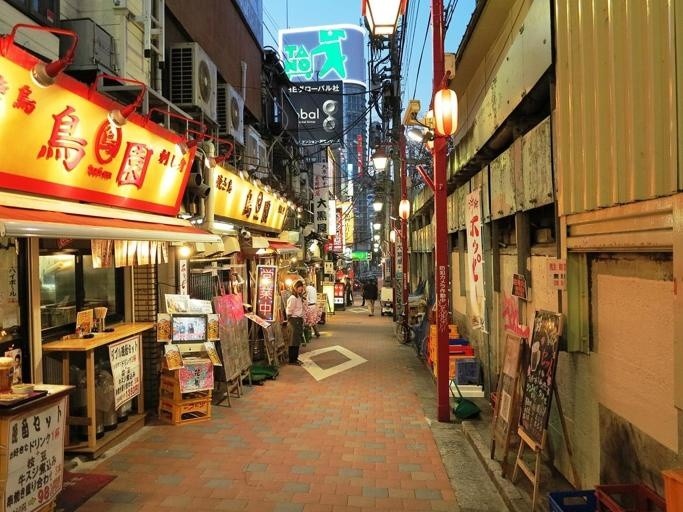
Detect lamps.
[230,153,303,213]
[0,21,233,170]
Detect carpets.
[54,464,118,511]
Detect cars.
[353,278,362,290]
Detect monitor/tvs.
[172,313,207,360]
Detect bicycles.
[395,302,416,343]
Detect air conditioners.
[216,83,245,147]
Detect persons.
[345,277,353,305]
[189,323,194,337]
[286,281,304,365]
[179,322,185,340]
[361,279,378,316]
[304,281,320,343]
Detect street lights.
[361,0,459,425]
[369,132,415,330]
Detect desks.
[42,320,155,452]
[0,382,77,512]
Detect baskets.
[428,324,480,380]
[550,484,665,512]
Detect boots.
[288,345,303,365]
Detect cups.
[0,356,13,391]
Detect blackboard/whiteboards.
[517,311,565,452]
